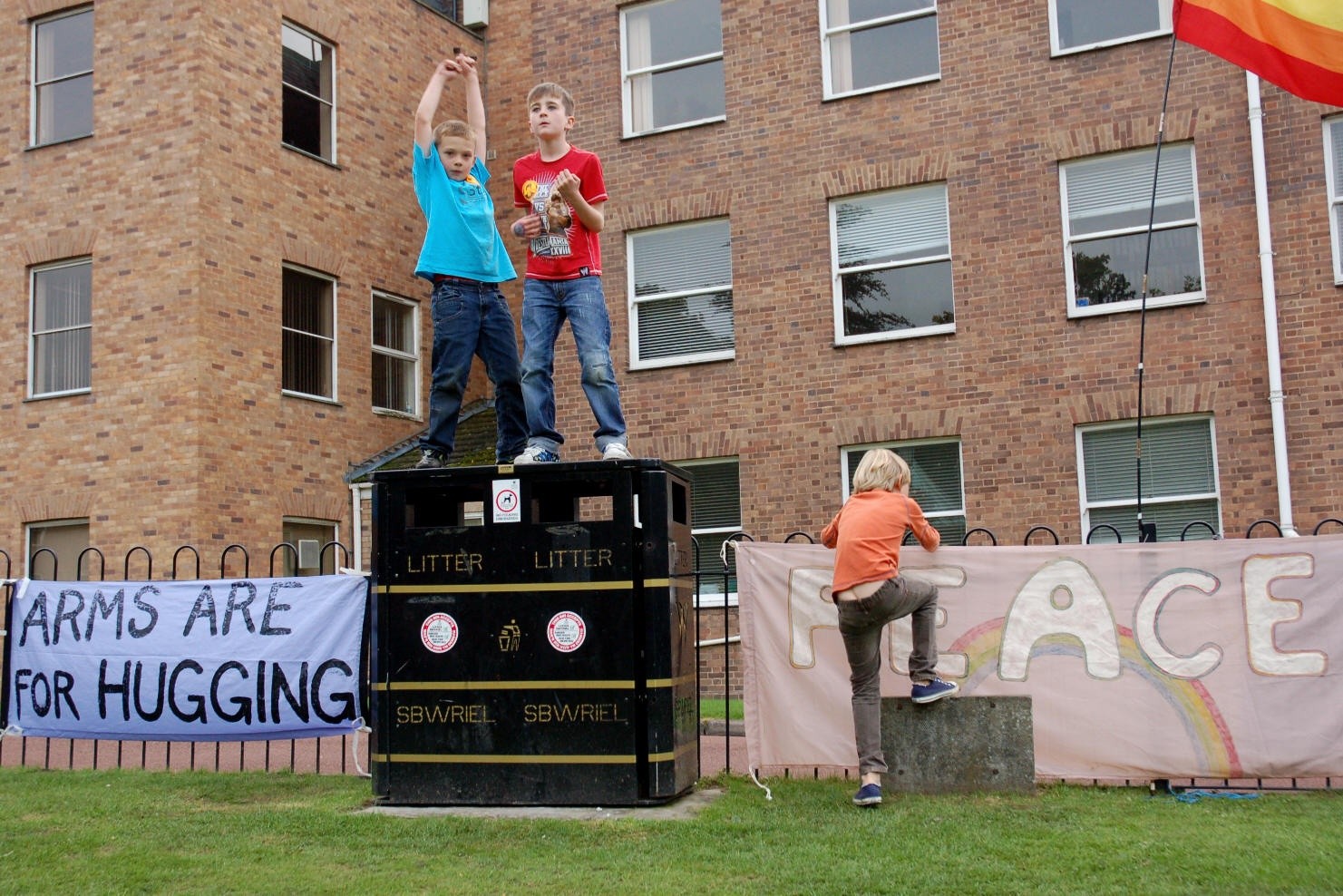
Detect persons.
[820,449,960,806]
[412,51,530,469]
[510,83,634,466]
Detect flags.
[1172,0,1343,107]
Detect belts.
[434,274,477,283]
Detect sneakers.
[604,443,633,459]
[416,449,450,468]
[852,783,882,805]
[514,447,561,464]
[495,449,522,464]
[911,676,958,704]
